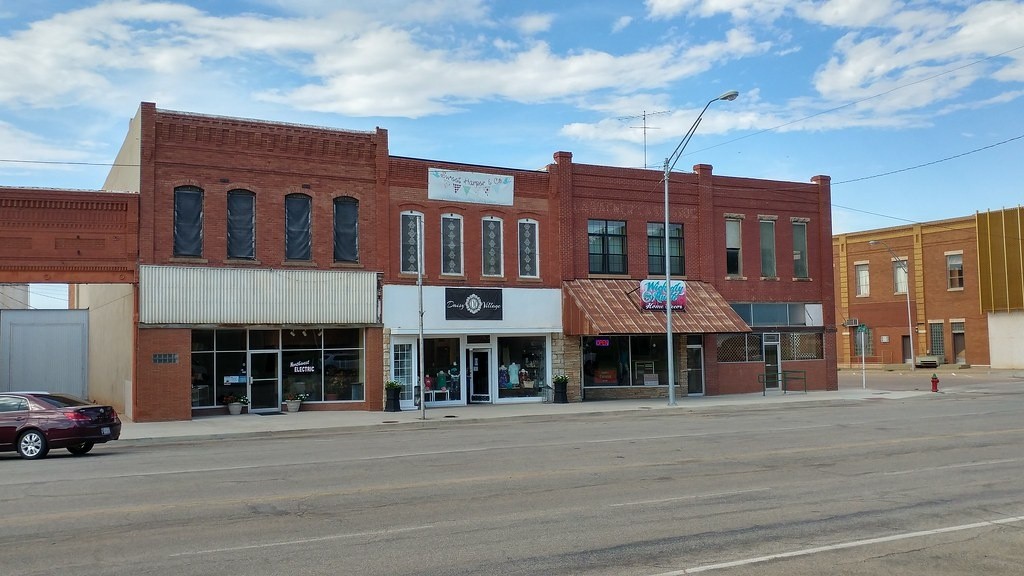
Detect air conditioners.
[845,318,858,326]
[881,336,889,342]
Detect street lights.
[869,240,918,372]
[662,90,741,402]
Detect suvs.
[317,354,358,377]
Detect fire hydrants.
[929,373,941,392]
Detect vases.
[327,393,337,400]
[285,400,301,412]
[383,388,402,412]
[553,382,568,403]
[228,403,243,415]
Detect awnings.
[137,265,384,326]
[561,280,755,336]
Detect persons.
[447,361,461,400]
[508,362,520,385]
[435,371,447,390]
[423,374,433,390]
[519,369,530,386]
[497,365,509,388]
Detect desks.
[426,390,450,402]
[499,387,521,398]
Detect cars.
[0,390,122,460]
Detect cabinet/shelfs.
[191,385,210,406]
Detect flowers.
[552,374,570,384]
[287,393,309,401]
[384,380,405,390]
[324,370,345,394]
[223,395,250,406]
